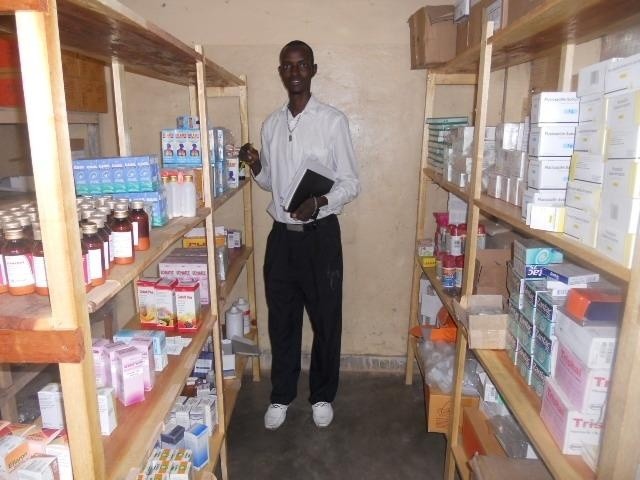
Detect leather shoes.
[262,403,290,430]
[312,402,334,428]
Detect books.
[282,166,335,220]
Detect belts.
[273,214,336,232]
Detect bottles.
[445,224,456,252]
[0,200,49,296]
[169,175,181,218]
[129,201,150,251]
[450,228,461,255]
[225,307,244,339]
[477,224,486,249]
[456,256,464,288]
[233,297,250,335]
[75,194,135,293]
[161,176,173,220]
[441,255,456,287]
[458,224,467,254]
[181,175,196,217]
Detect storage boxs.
[407,4,457,70]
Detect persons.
[240,40,362,429]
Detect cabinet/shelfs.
[405,0,640,480]
[0,0,260,480]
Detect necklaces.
[286,104,305,141]
